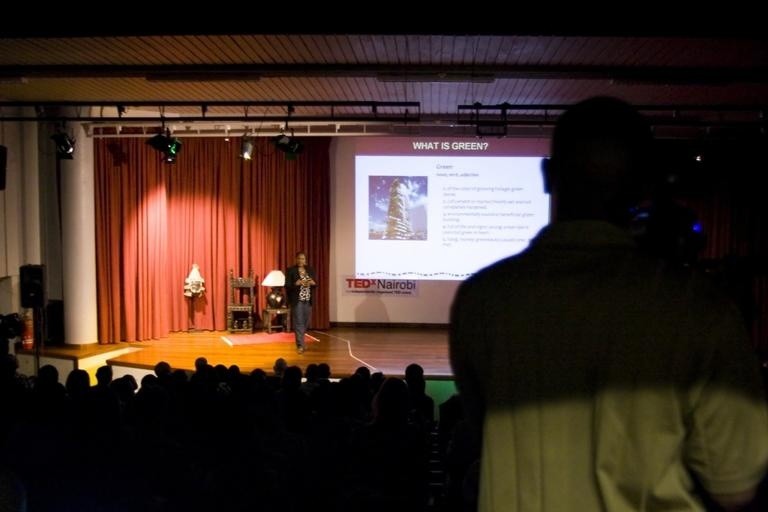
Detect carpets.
[220,330,320,348]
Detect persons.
[284,250,320,356]
[444,91,768,511]
[0,352,435,512]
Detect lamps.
[260,269,287,307]
[47,124,306,163]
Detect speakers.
[19,265,50,308]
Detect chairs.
[226,267,258,333]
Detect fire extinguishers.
[22,308,34,350]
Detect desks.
[261,308,292,336]
